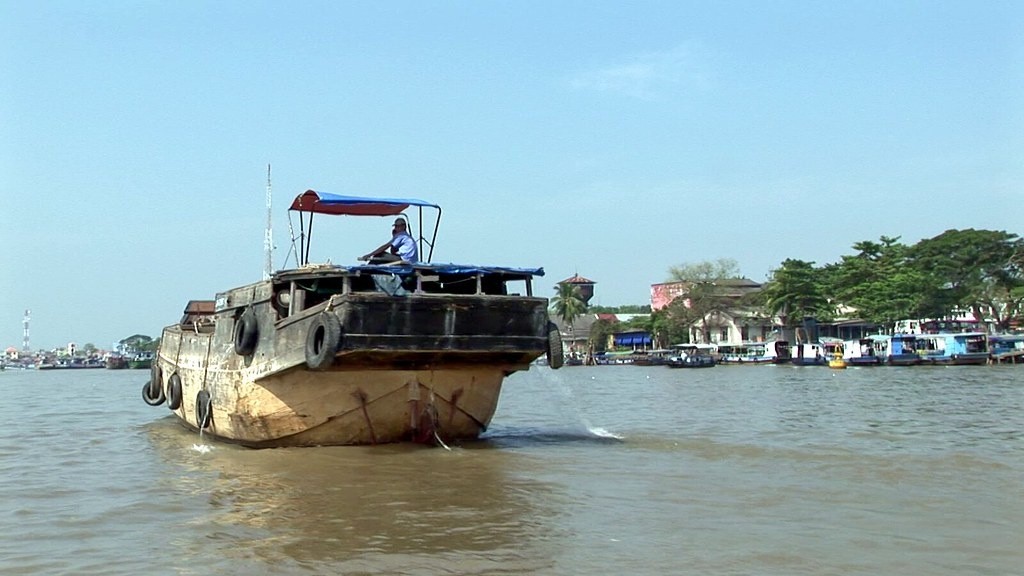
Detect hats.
[392,218,406,226]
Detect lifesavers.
[166,374,182,410]
[195,390,211,428]
[270,281,291,314]
[150,365,161,399]
[304,310,340,370]
[547,322,564,369]
[234,313,258,356]
[142,380,166,407]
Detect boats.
[126,354,153,371]
[141,164,566,448]
[103,353,124,370]
[790,333,1024,376]
[721,339,790,364]
[670,343,723,369]
[629,348,676,367]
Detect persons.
[364,218,418,264]
